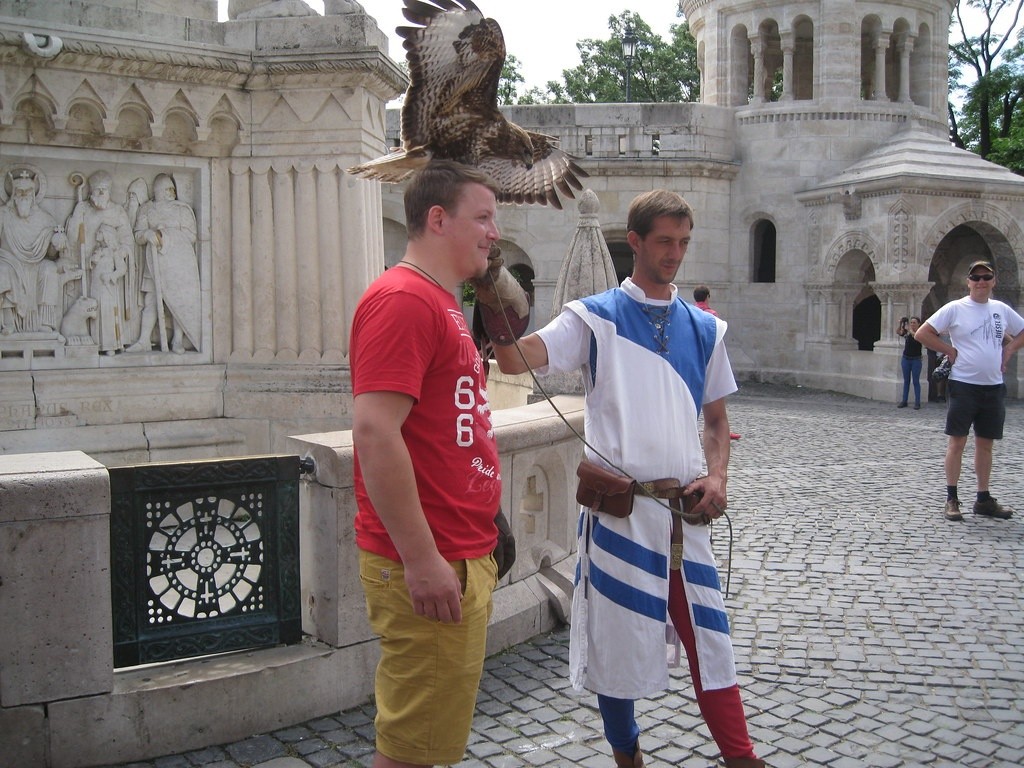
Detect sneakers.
[972,497,1014,519]
[944,498,963,521]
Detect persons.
[896,316,922,409]
[469,190,768,768]
[0,171,201,353]
[351,161,503,768]
[693,286,741,439]
[914,261,1024,521]
[932,355,951,397]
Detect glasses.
[969,274,993,281]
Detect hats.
[967,257,994,276]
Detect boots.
[612,738,647,768]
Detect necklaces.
[400,261,443,288]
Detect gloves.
[492,507,518,580]
[468,242,531,347]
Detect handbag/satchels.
[575,461,637,518]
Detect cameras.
[900,317,909,322]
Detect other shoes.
[897,402,907,408]
[914,404,920,410]
[934,395,946,403]
